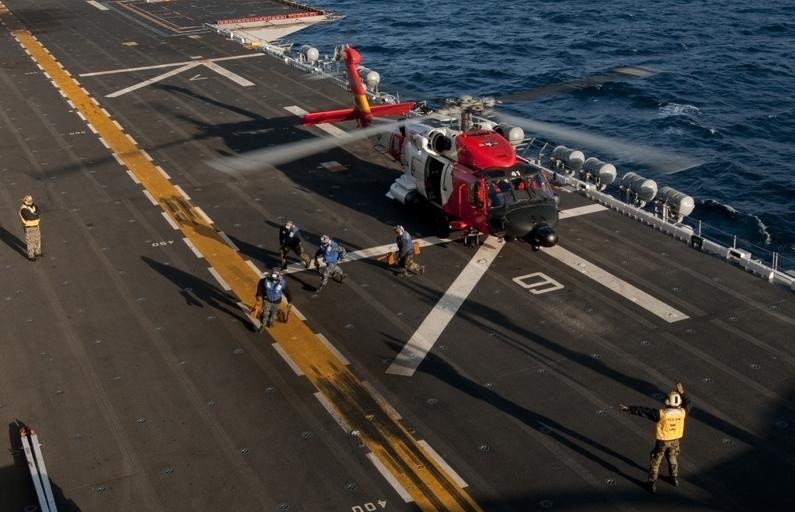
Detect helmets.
[285,221,293,230]
[320,235,329,246]
[664,391,683,407]
[270,270,280,281]
[393,224,404,236]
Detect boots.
[671,476,680,487]
[316,284,326,293]
[305,259,312,268]
[340,273,347,284]
[649,480,657,493]
[258,326,265,334]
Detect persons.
[17,193,44,263]
[475,182,501,208]
[517,176,540,190]
[393,223,425,278]
[618,380,693,493]
[315,234,348,298]
[278,219,311,270]
[255,270,292,334]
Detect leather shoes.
[36,252,47,257]
[29,257,39,262]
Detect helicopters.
[205,44,662,253]
